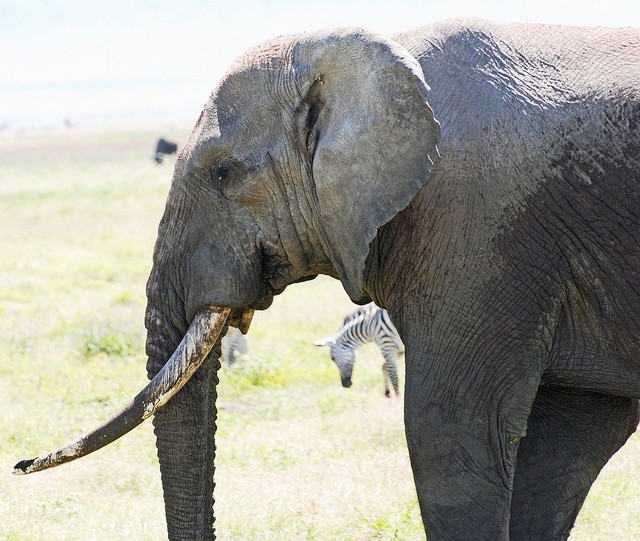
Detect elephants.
[12,18,640,540]
